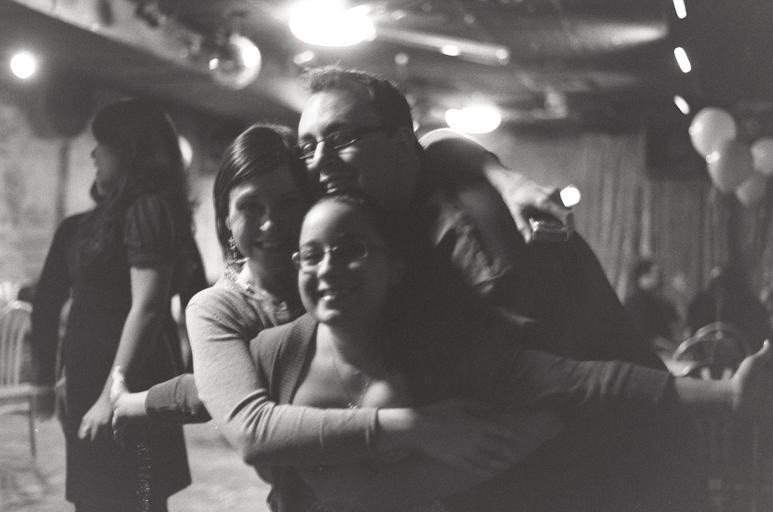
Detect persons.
[688,260,770,368]
[182,120,578,511]
[107,187,772,510]
[283,62,706,512]
[25,187,210,425]
[614,256,680,362]
[45,92,202,511]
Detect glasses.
[300,128,359,159]
[291,242,368,264]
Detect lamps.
[208,5,262,90]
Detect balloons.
[703,139,751,190]
[686,106,739,155]
[750,137,773,176]
[731,168,767,206]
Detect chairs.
[0,299,36,453]
[674,321,752,503]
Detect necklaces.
[319,328,379,412]
